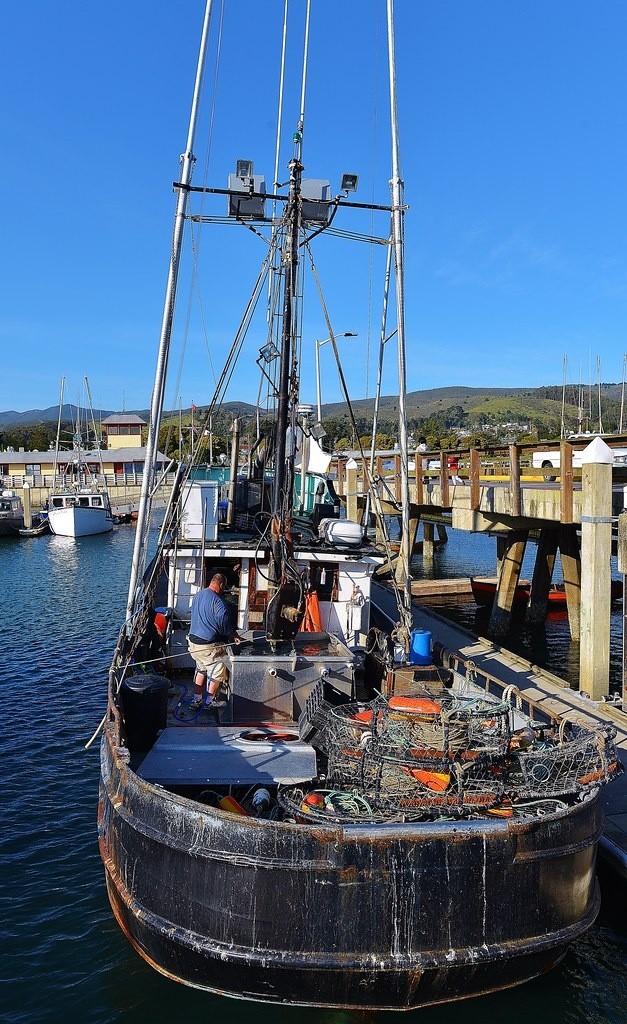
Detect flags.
[192,404,197,411]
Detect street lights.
[314,330,358,448]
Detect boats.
[469,573,623,609]
[0,490,24,536]
[78,0,607,1015]
[46,374,114,537]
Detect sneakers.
[189,699,202,710]
[203,698,227,710]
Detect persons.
[188,574,242,710]
[447,454,465,486]
[415,437,431,484]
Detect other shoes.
[462,480,466,486]
[424,476,429,484]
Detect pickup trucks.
[531,433,627,483]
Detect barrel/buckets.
[120,674,174,740]
[410,629,434,666]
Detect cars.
[380,460,441,471]
